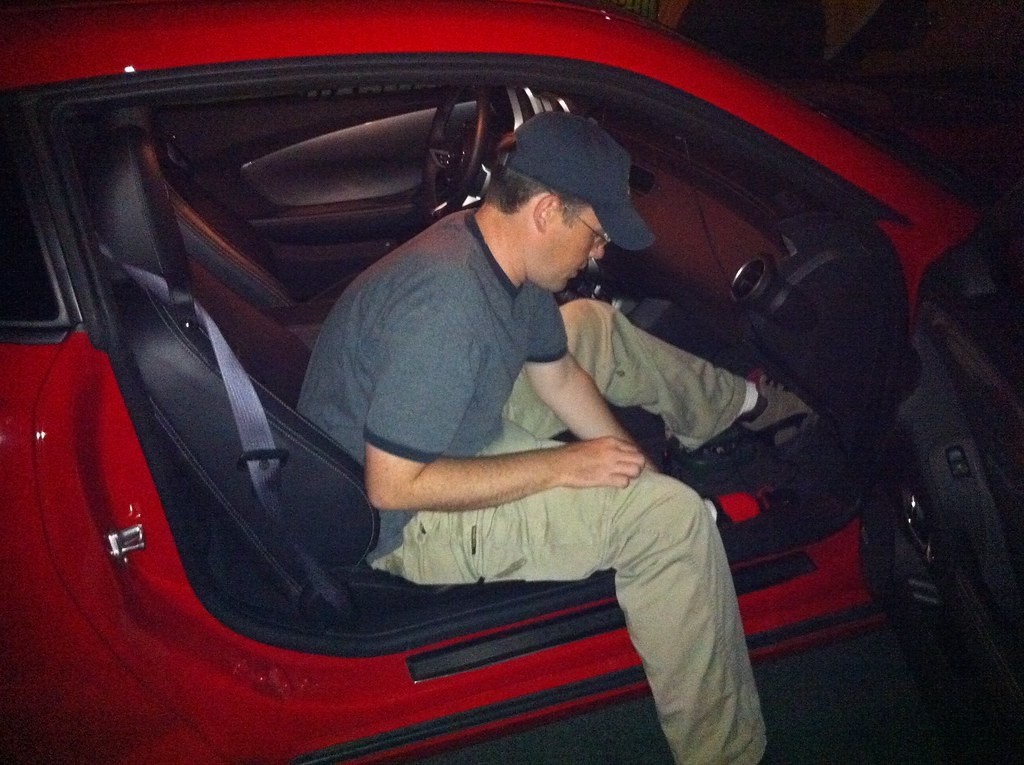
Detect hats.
[499,111,657,251]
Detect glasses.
[545,189,612,251]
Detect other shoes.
[743,376,810,443]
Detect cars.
[0,0,1022,765]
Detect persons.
[296,112,819,765]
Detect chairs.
[86,104,523,637]
